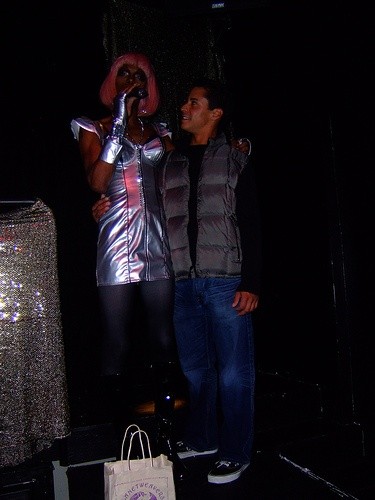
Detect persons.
[74,53,250,457]
[91,81,263,485]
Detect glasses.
[119,69,147,82]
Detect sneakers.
[176,441,219,458]
[207,460,251,484]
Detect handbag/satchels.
[103,424,176,500]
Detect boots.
[153,393,178,458]
[114,391,144,459]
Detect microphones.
[127,83,145,98]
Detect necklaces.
[113,113,145,150]
